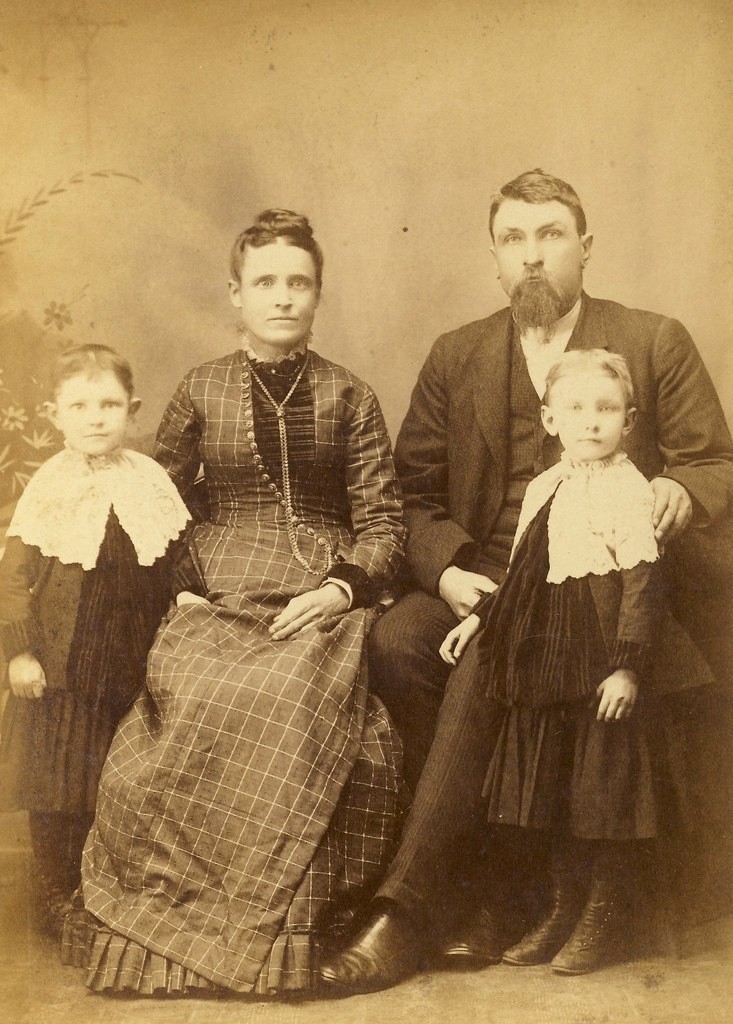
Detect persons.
[319,168,733,994]
[438,349,723,975]
[0,343,211,937]
[58,210,413,996]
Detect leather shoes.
[319,903,423,994]
[438,902,523,963]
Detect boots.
[501,866,589,967]
[549,870,634,975]
[29,859,84,940]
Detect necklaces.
[244,355,332,575]
